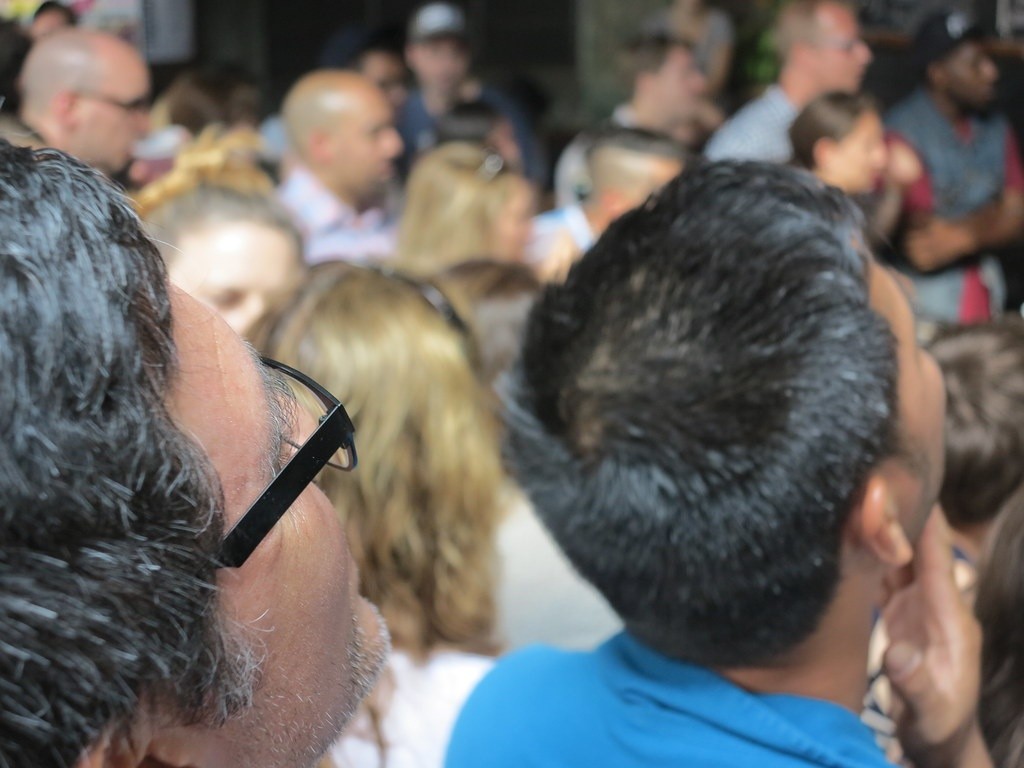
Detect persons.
[0,0,1024,768]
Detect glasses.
[76,89,151,112]
[204,353,358,569]
[369,264,474,338]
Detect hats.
[911,14,981,70]
[406,2,469,45]
